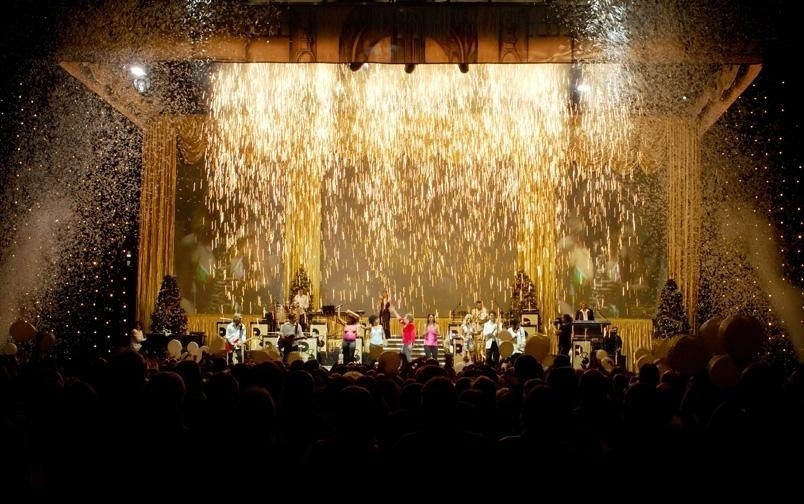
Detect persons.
[0,288,804,504]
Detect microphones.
[335,304,343,309]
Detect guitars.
[278,335,309,349]
[225,337,256,353]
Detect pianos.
[140,333,203,362]
